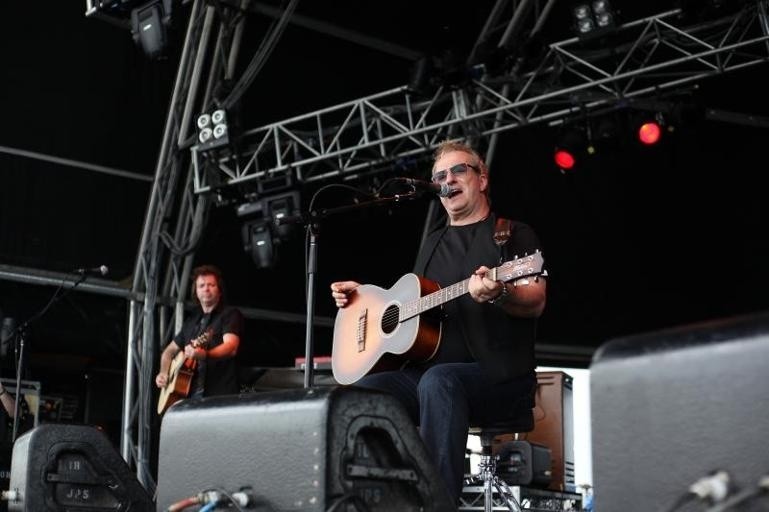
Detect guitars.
[331,248,546,387]
[157,328,210,414]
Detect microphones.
[404,181,453,196]
[74,265,109,276]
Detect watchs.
[488,281,509,309]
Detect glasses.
[431,163,479,182]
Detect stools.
[458,407,535,511]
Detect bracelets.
[0,389,6,395]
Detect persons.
[156,265,245,397]
[0,383,30,512]
[330,144,547,508]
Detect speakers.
[7,423,155,512]
[572,314,769,512]
[155,382,461,511]
